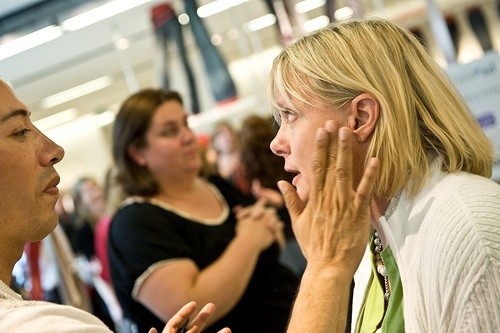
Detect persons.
[0,78,380,333]
[266,18,500,333]
